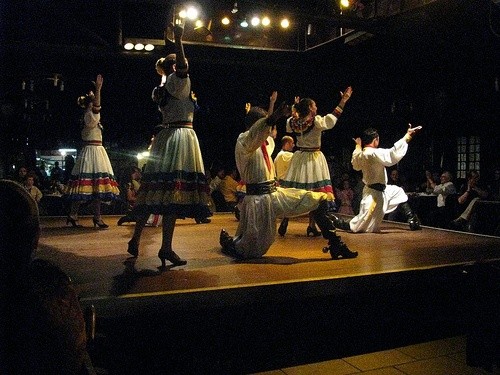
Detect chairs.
[446,193,466,231]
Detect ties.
[27,189,31,193]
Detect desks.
[414,193,438,218]
[471,199,500,235]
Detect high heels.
[66,215,82,228]
[275,217,291,236]
[127,239,139,257]
[93,217,109,228]
[158,248,187,266]
[306,225,321,236]
[232,206,241,221]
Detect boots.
[117,216,128,225]
[328,213,351,229]
[195,215,211,224]
[220,228,234,253]
[328,237,358,260]
[406,211,422,232]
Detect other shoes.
[449,220,464,229]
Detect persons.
[66,74,121,229]
[0,85,500,260]
[127,19,217,265]
[0,179,95,375]
